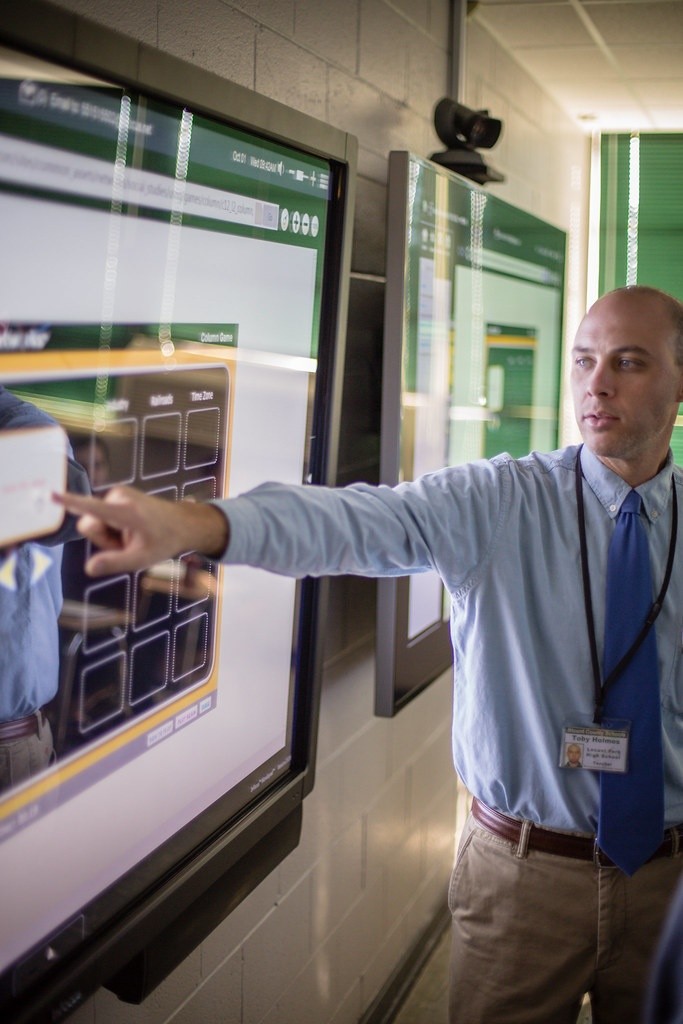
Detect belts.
[471,796,683,868]
[0,706,46,744]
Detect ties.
[595,489,665,876]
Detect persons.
[566,743,582,768]
[65,432,131,727]
[51,287,683,1024]
[0,385,95,792]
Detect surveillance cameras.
[436,99,503,149]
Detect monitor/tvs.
[375,150,566,717]
[0,0,358,1024]
